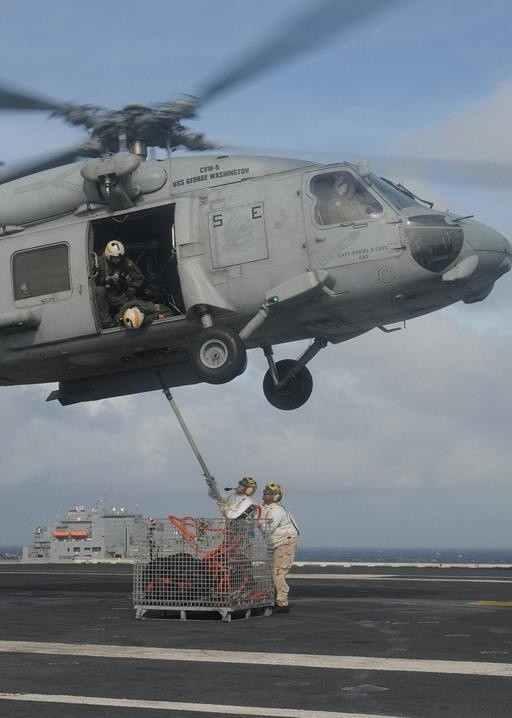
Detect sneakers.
[274,606,289,613]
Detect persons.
[95,239,145,330]
[327,173,377,222]
[114,299,174,330]
[201,472,258,532]
[257,481,298,614]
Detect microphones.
[224,488,237,491]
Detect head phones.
[273,494,282,502]
[245,487,254,496]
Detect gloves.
[203,473,216,487]
[218,503,227,512]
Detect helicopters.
[1,0,512,409]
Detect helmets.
[239,477,283,503]
[122,306,145,330]
[104,240,125,261]
